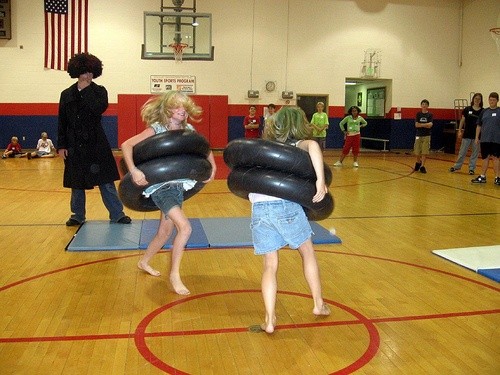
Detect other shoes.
[471,176,487,183]
[117,216,132,224]
[494,177,500,185]
[353,161,359,167]
[27,153,31,160]
[414,160,422,171]
[420,166,426,173]
[65,218,79,226]
[332,160,343,166]
[2,154,7,158]
[469,170,474,175]
[448,166,461,172]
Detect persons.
[120,90,217,296]
[244,101,368,166]
[248,105,330,334]
[2,136,28,158]
[450,93,484,175]
[472,92,500,185]
[27,132,59,159]
[56,52,132,226]
[415,99,433,173]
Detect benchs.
[361,136,389,151]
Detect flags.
[44,0,88,71]
